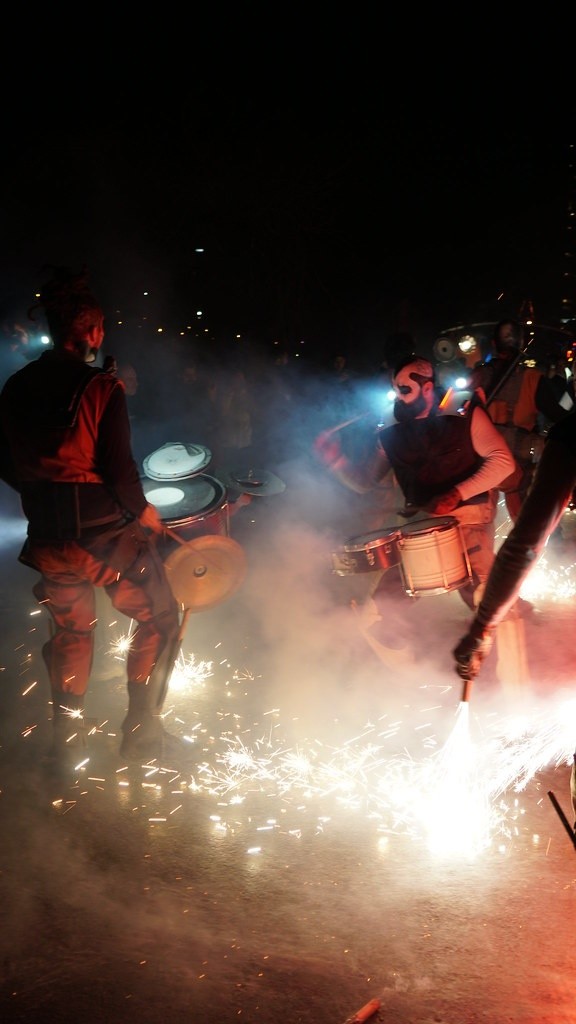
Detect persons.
[111,306,576,708]
[2,292,199,783]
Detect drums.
[133,475,228,558]
[340,529,401,574]
[397,516,473,599]
[143,442,214,483]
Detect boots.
[119,682,200,762]
[47,690,88,778]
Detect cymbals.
[162,535,250,612]
[222,468,286,497]
